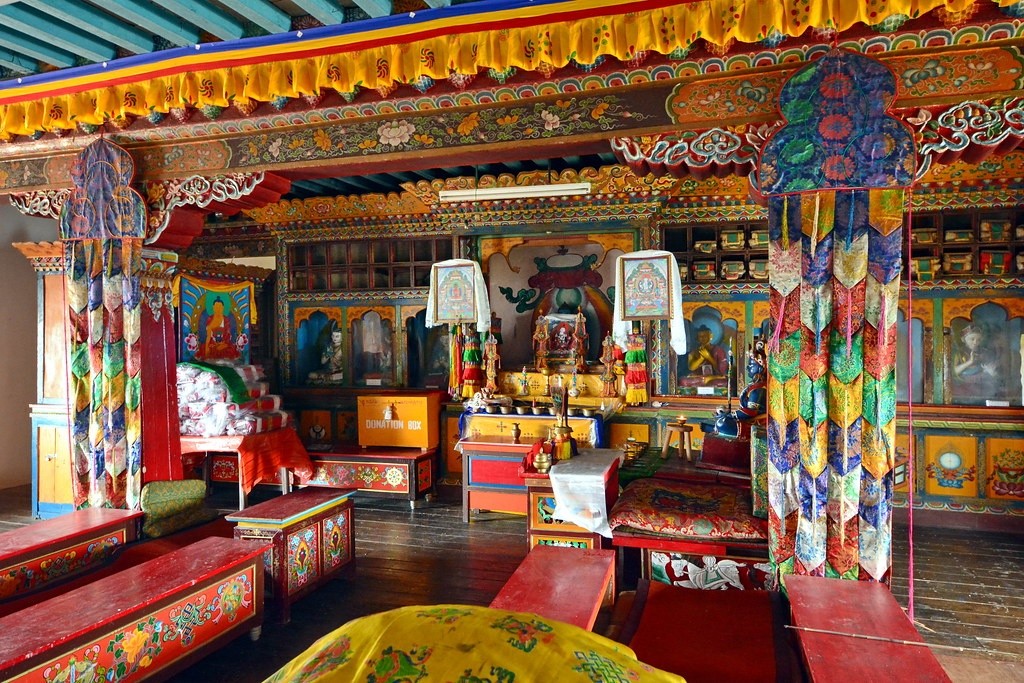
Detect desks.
[461,410,603,448]
[178,426,293,510]
[211,444,438,509]
[457,434,587,523]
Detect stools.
[661,424,693,462]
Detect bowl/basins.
[531,407,546,415]
[547,406,557,416]
[499,406,513,414]
[582,407,597,416]
[567,407,580,416]
[514,405,531,415]
[486,405,497,414]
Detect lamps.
[439,182,591,203]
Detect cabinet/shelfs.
[661,221,769,284]
[900,207,1024,278]
[223,487,360,626]
[518,449,625,584]
[287,236,454,292]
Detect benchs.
[487,543,617,631]
[780,572,952,683]
[0,536,275,683]
[0,508,145,604]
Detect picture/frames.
[433,263,478,323]
[619,254,674,321]
